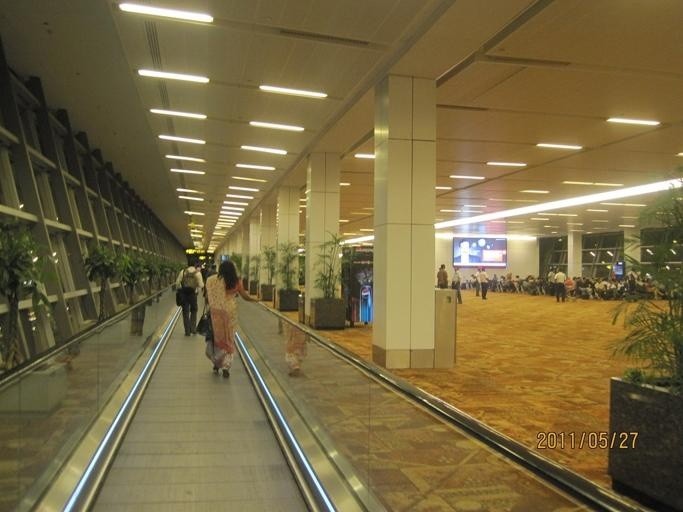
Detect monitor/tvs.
[452,237,508,268]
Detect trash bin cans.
[298,292,305,323]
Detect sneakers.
[213,366,229,377]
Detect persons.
[174,256,206,338]
[283,326,311,374]
[203,259,262,377]
[434,262,682,305]
[453,239,480,264]
[195,261,218,306]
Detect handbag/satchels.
[176,286,196,305]
[196,313,210,335]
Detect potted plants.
[0,217,186,410]
[241,230,353,329]
[604,163,681,512]
